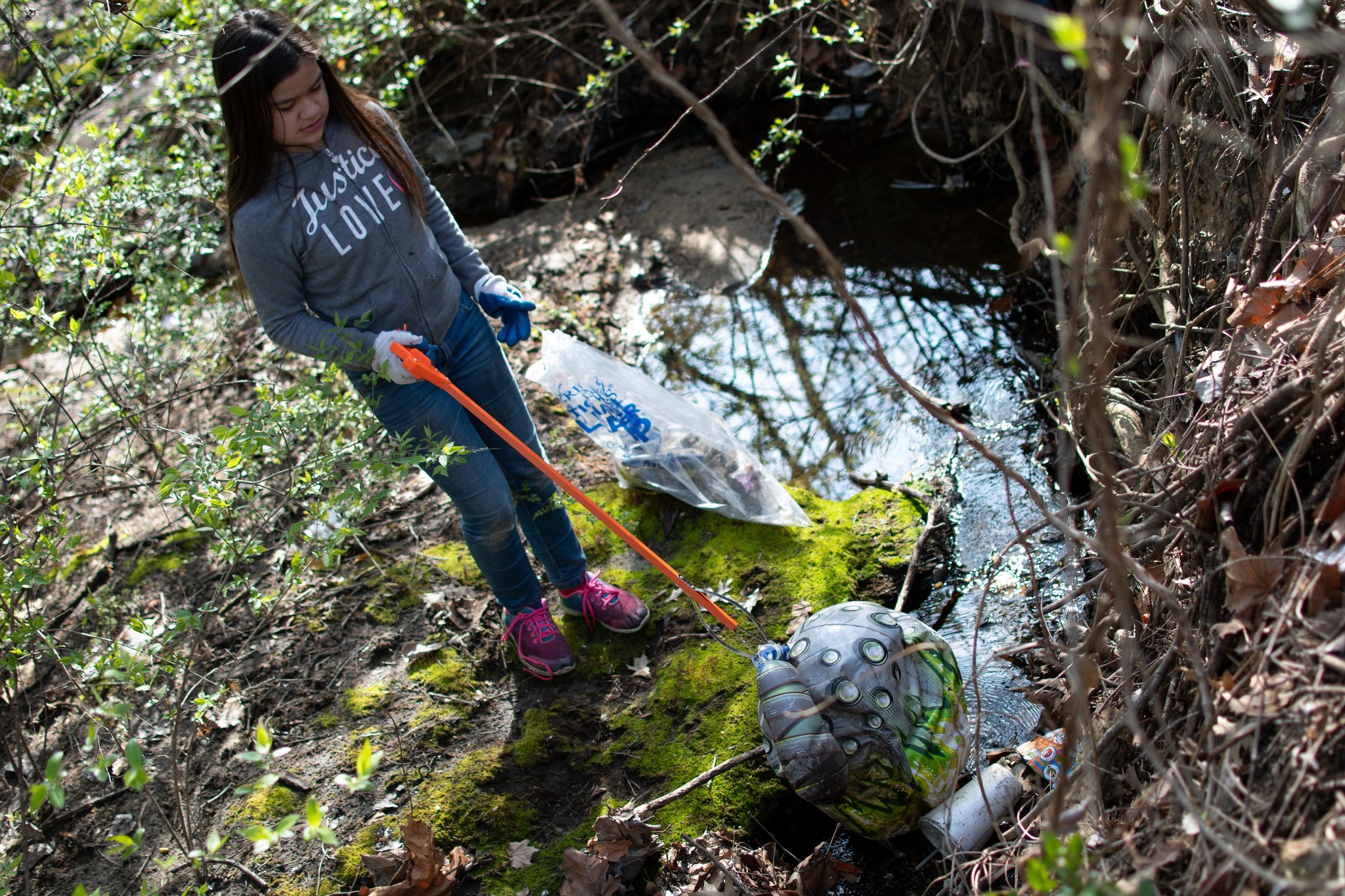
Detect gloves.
[371,330,441,386]
[479,275,536,346]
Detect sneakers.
[501,599,576,680]
[558,570,650,634]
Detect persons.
[214,7,650,674]
